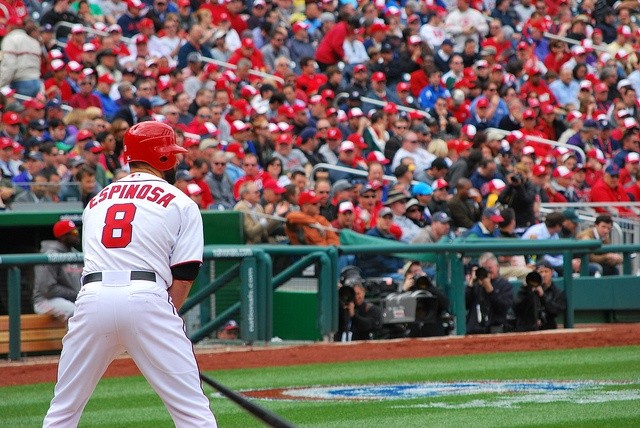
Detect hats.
[50,59,65,74]
[84,140,105,152]
[298,191,324,206]
[383,190,412,206]
[322,127,342,140]
[326,108,337,119]
[348,107,364,120]
[108,24,122,33]
[533,166,548,177]
[599,119,611,130]
[407,111,425,122]
[349,89,362,102]
[455,79,475,89]
[0,137,13,149]
[23,99,45,110]
[2,111,23,125]
[553,147,572,158]
[226,142,246,159]
[200,63,220,80]
[477,99,489,108]
[333,179,356,193]
[240,86,260,97]
[397,82,411,93]
[222,70,241,83]
[13,141,25,154]
[432,178,448,191]
[98,72,116,84]
[155,0,167,5]
[476,60,490,69]
[605,164,619,176]
[528,66,541,77]
[347,134,368,149]
[617,25,633,39]
[408,35,422,45]
[462,124,476,139]
[354,64,366,73]
[480,46,496,56]
[517,41,529,50]
[552,165,573,179]
[359,184,376,194]
[292,21,310,33]
[371,72,386,82]
[183,138,200,148]
[339,201,353,213]
[127,0,145,9]
[66,61,84,75]
[506,128,526,145]
[383,102,398,114]
[600,53,611,66]
[523,110,536,120]
[65,155,86,169]
[253,0,267,8]
[594,82,608,94]
[489,179,506,194]
[200,122,221,136]
[367,151,389,164]
[615,50,633,61]
[563,209,586,223]
[135,96,152,110]
[77,129,96,143]
[230,120,251,136]
[341,141,354,152]
[622,117,638,129]
[625,152,640,164]
[483,207,504,222]
[277,134,293,145]
[83,43,95,52]
[218,13,229,22]
[573,46,586,56]
[78,68,96,86]
[92,22,108,32]
[432,211,451,224]
[586,148,606,164]
[46,96,61,111]
[97,48,118,65]
[71,24,85,34]
[53,220,76,238]
[48,49,64,63]
[307,95,328,107]
[185,183,205,197]
[187,52,201,64]
[580,119,598,132]
[28,119,48,129]
[25,151,45,162]
[321,89,335,100]
[242,38,254,48]
[232,98,256,116]
[463,68,477,81]
[379,207,393,219]
[49,118,66,129]
[337,110,348,123]
[136,34,148,44]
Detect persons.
[465,252,514,335]
[385,261,448,338]
[40,121,220,428]
[31,219,84,328]
[339,277,384,340]
[216,319,240,339]
[1,0,640,275]
[511,261,567,332]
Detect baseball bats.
[199,371,295,427]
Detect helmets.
[123,121,187,185]
